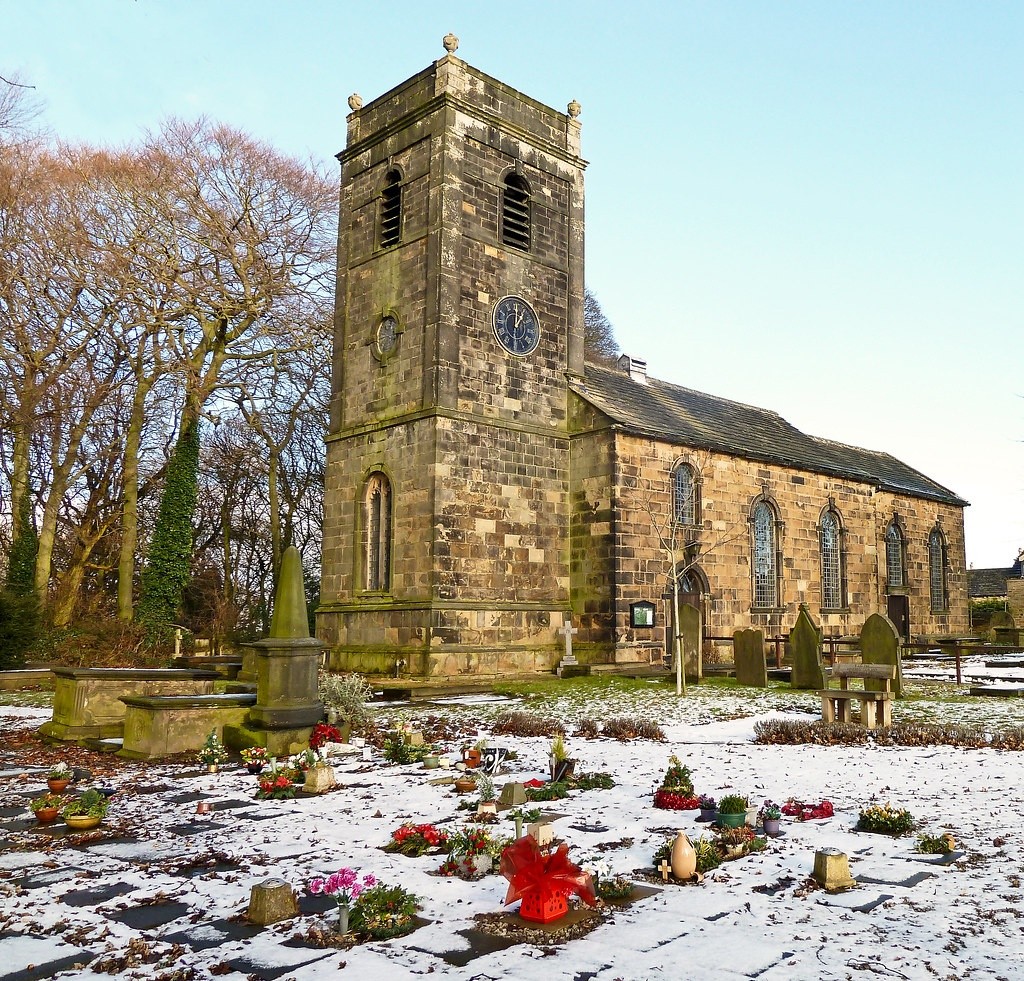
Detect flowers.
[758,799,781,820]
[311,867,377,904]
[195,727,228,763]
[697,793,716,808]
[240,747,269,766]
[440,826,499,875]
[475,772,499,802]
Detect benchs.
[814,662,897,729]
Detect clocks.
[493,295,541,357]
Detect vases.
[670,832,696,878]
[207,762,218,773]
[247,761,263,774]
[339,904,349,936]
[700,806,717,820]
[455,854,492,875]
[455,781,478,791]
[763,819,780,835]
[422,756,439,768]
[477,802,496,814]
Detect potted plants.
[546,736,578,782]
[31,797,62,821]
[62,790,110,828]
[47,771,70,793]
[714,794,748,829]
[722,827,755,853]
[468,739,486,764]
